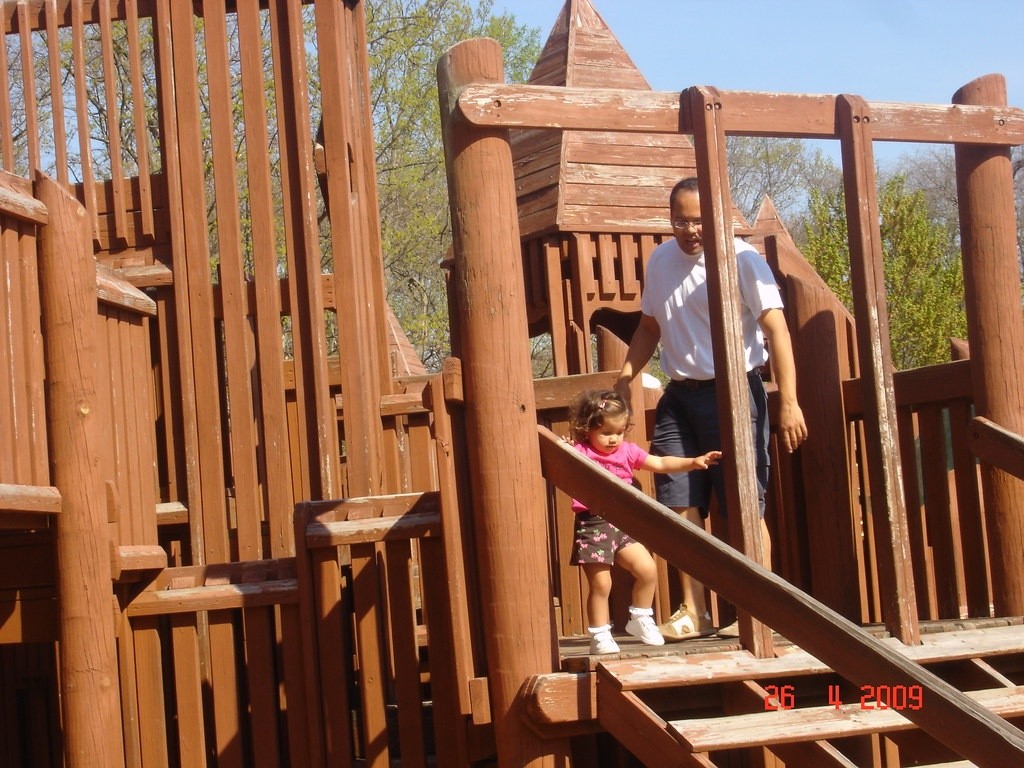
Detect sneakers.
[590,632,620,654]
[625,617,665,646]
[656,604,717,642]
[717,617,781,637]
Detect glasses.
[672,217,703,230]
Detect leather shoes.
[671,367,760,390]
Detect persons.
[613,178,808,641]
[558,390,722,654]
